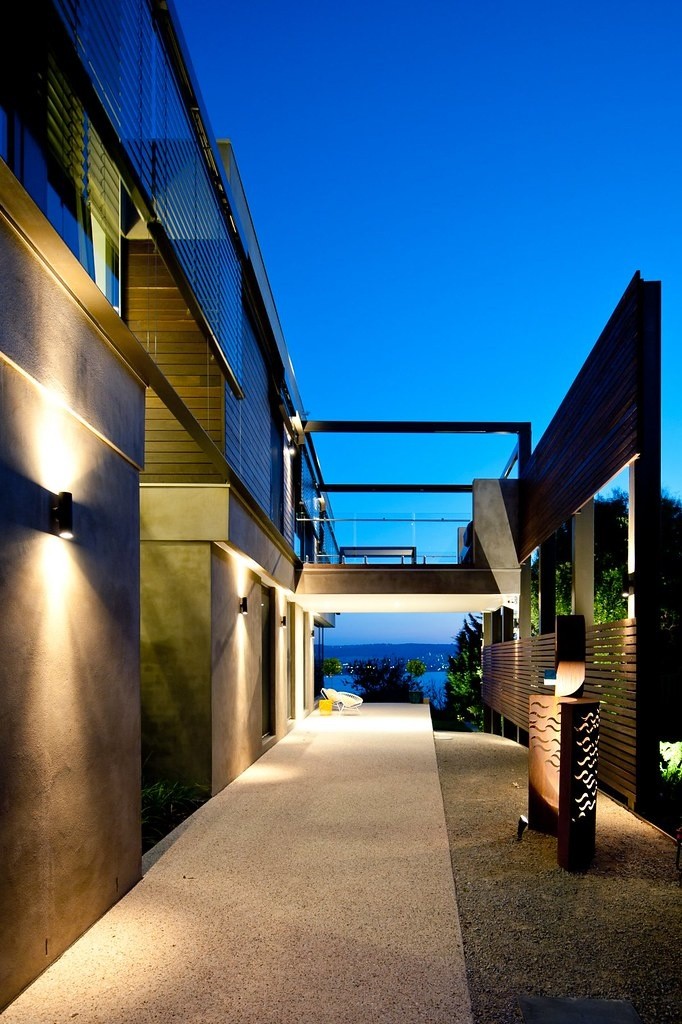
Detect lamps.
[239,597,248,615]
[281,616,286,626]
[49,491,74,540]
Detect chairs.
[320,687,363,717]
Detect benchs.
[339,546,417,564]
